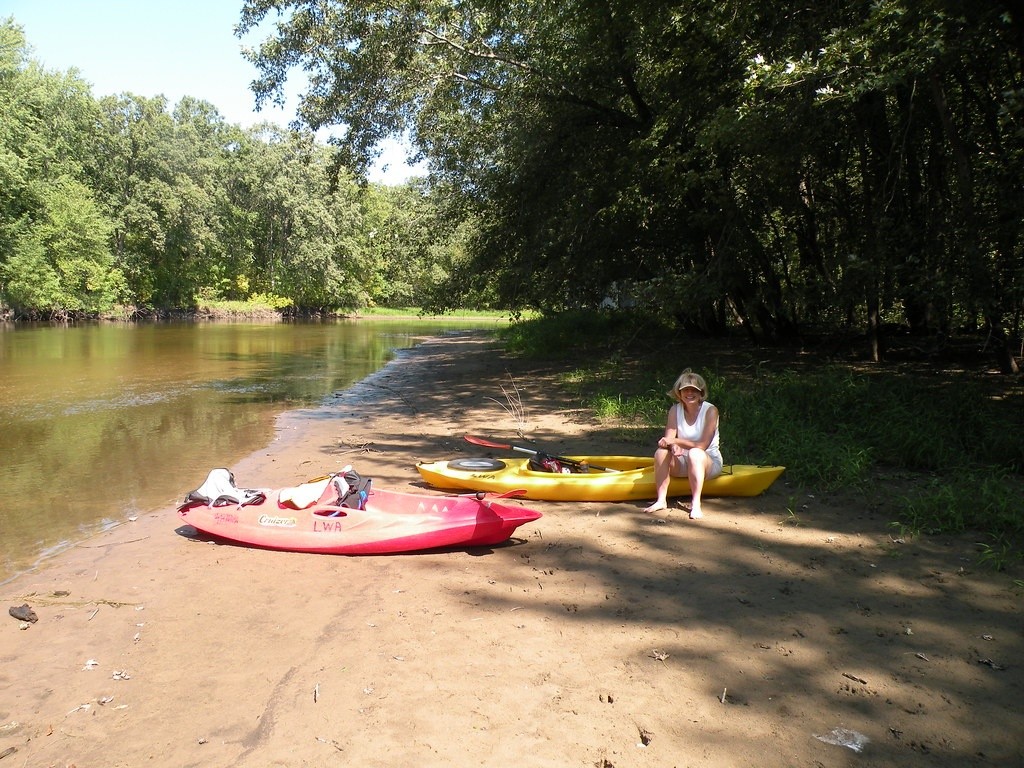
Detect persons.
[643,372,723,520]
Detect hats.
[679,374,705,392]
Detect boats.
[176,463,543,555]
[416,435,787,502]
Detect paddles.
[462,432,621,473]
